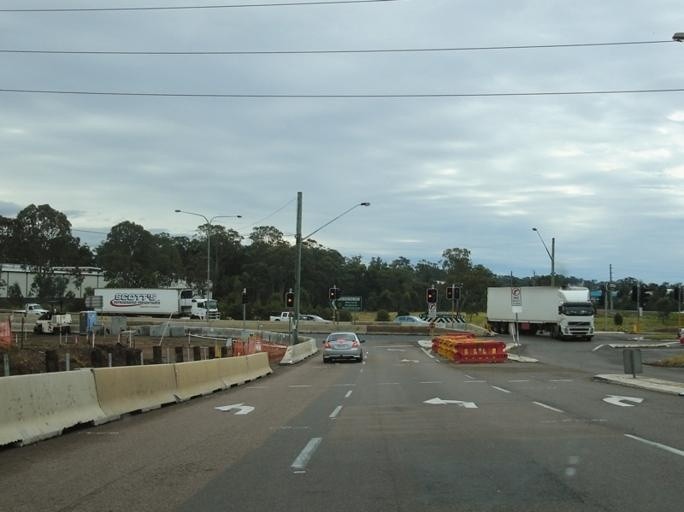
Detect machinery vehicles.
[33,300,73,335]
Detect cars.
[300,315,323,320]
[321,332,365,362]
[393,315,423,323]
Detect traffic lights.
[454,287,460,299]
[336,288,343,299]
[328,288,335,299]
[426,288,437,303]
[446,287,451,299]
[241,293,248,304]
[286,293,294,307]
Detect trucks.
[91,287,220,321]
[11,303,50,322]
[485,285,596,342]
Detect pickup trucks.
[269,311,294,323]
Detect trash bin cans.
[623,350,642,377]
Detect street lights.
[532,227,555,285]
[291,192,371,344]
[173,209,242,323]
[672,32,684,41]
